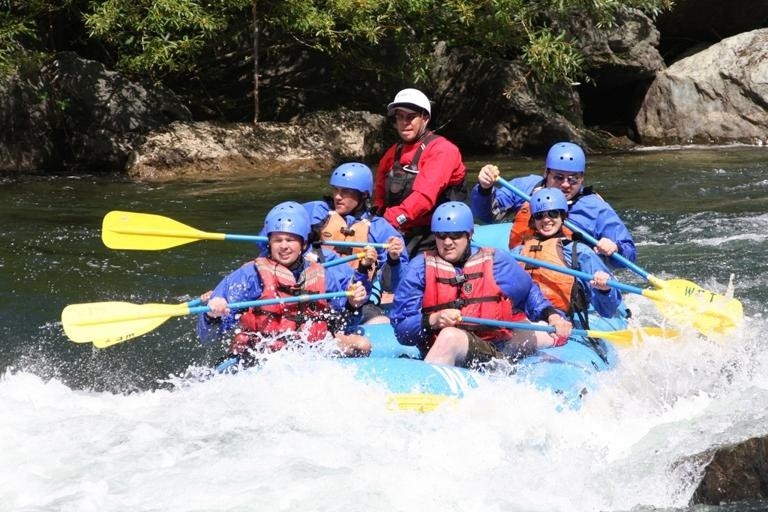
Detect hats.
[386,106,418,115]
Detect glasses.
[549,170,582,185]
[534,209,560,220]
[435,230,464,240]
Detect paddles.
[100,210,392,251]
[471,242,744,332]
[455,316,683,355]
[494,174,744,344]
[60,291,352,345]
[92,251,365,349]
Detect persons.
[255,163,410,324]
[496,188,622,359]
[468,141,636,269]
[373,88,469,259]
[389,201,571,370]
[198,201,373,364]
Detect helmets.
[330,163,373,199]
[546,142,584,172]
[387,88,430,120]
[529,188,568,216]
[264,201,311,240]
[432,202,474,237]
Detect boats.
[223,218,633,416]
